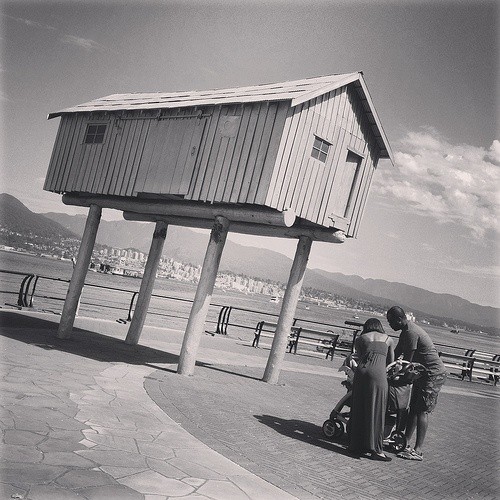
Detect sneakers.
[395,445,424,460]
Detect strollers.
[321,354,424,454]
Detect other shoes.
[371,453,393,461]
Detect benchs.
[252,321,340,361]
[438,352,500,386]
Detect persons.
[346,318,394,462]
[386,306,448,461]
